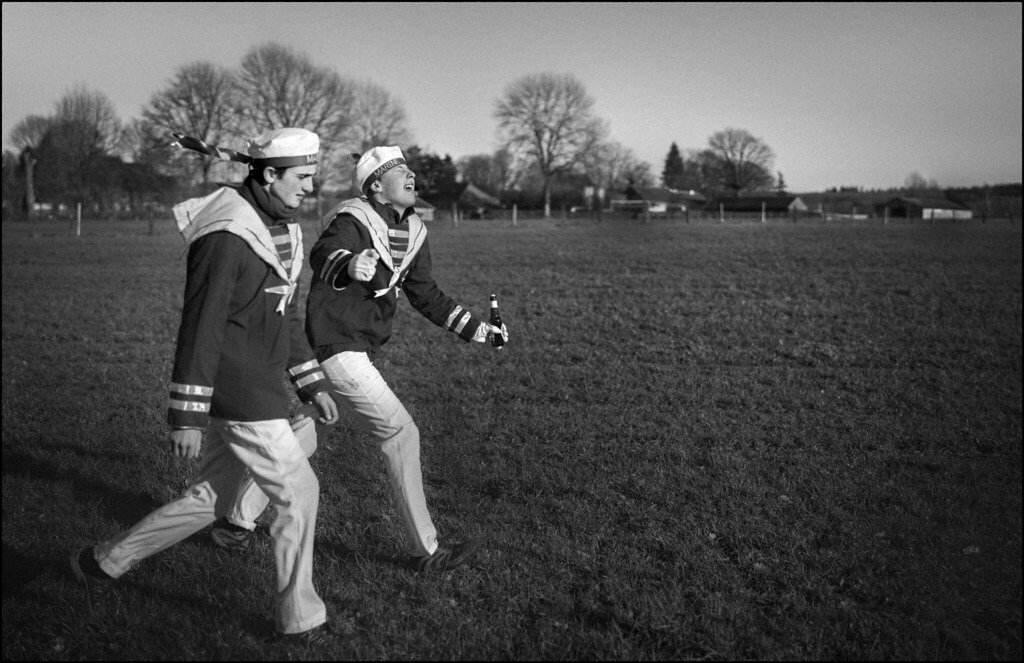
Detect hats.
[170,127,320,169]
[354,145,407,196]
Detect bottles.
[488,293,504,351]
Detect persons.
[80,127,339,646]
[210,144,509,570]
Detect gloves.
[473,323,511,349]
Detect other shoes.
[281,622,362,655]
[68,546,120,623]
[409,542,476,575]
[209,517,271,554]
